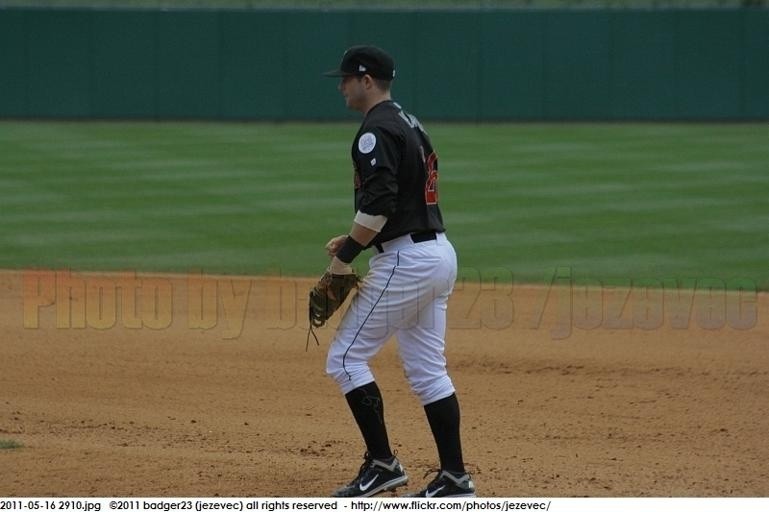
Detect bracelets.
[335,236,365,265]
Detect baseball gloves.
[310,269,357,327]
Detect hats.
[322,43,397,79]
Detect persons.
[307,45,477,498]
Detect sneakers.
[401,470,476,497]
[331,454,409,497]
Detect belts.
[375,231,439,253]
